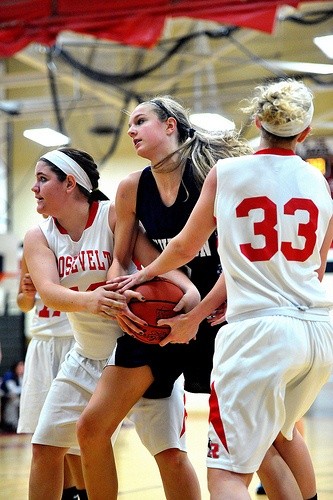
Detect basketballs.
[119,274,185,346]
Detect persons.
[105,78,333,500]
[76,95,303,500]
[21,147,203,500]
[17,253,90,500]
